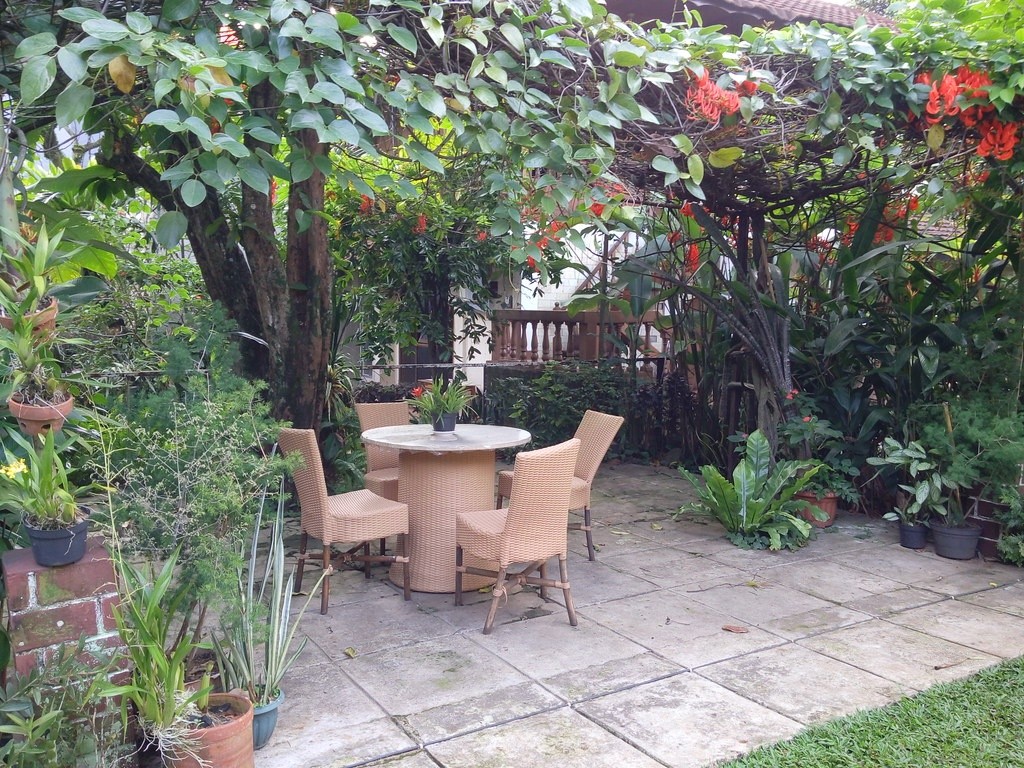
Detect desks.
[361,424,531,593]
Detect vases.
[792,491,839,529]
[0,214,85,343]
[22,505,91,567]
[432,412,455,431]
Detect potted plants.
[108,488,335,768]
[865,434,990,559]
[8,306,73,434]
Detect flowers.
[404,371,478,416]
[0,424,90,526]
[727,387,865,506]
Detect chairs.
[276,426,411,615]
[455,437,581,635]
[355,400,410,564]
[497,410,625,561]
